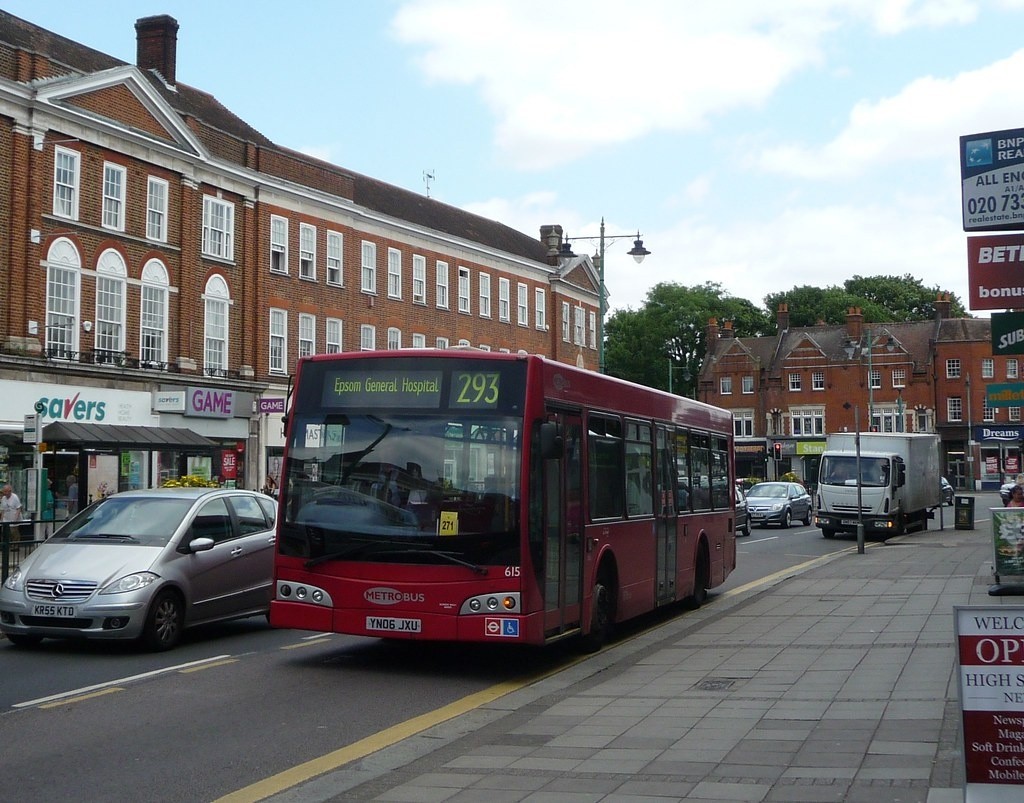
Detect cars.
[0,484,282,651]
[745,481,814,529]
[734,485,752,536]
[940,476,955,506]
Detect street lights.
[840,323,895,552]
[667,358,692,393]
[554,217,651,380]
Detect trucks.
[813,430,943,537]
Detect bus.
[268,347,739,647]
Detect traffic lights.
[869,424,878,432]
[761,449,769,462]
[774,443,783,461]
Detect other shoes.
[11,546,20,552]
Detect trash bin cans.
[954,494,975,529]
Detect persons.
[829,464,847,482]
[0,485,25,551]
[269,458,282,489]
[41,474,78,538]
[262,475,276,499]
[1005,485,1024,508]
